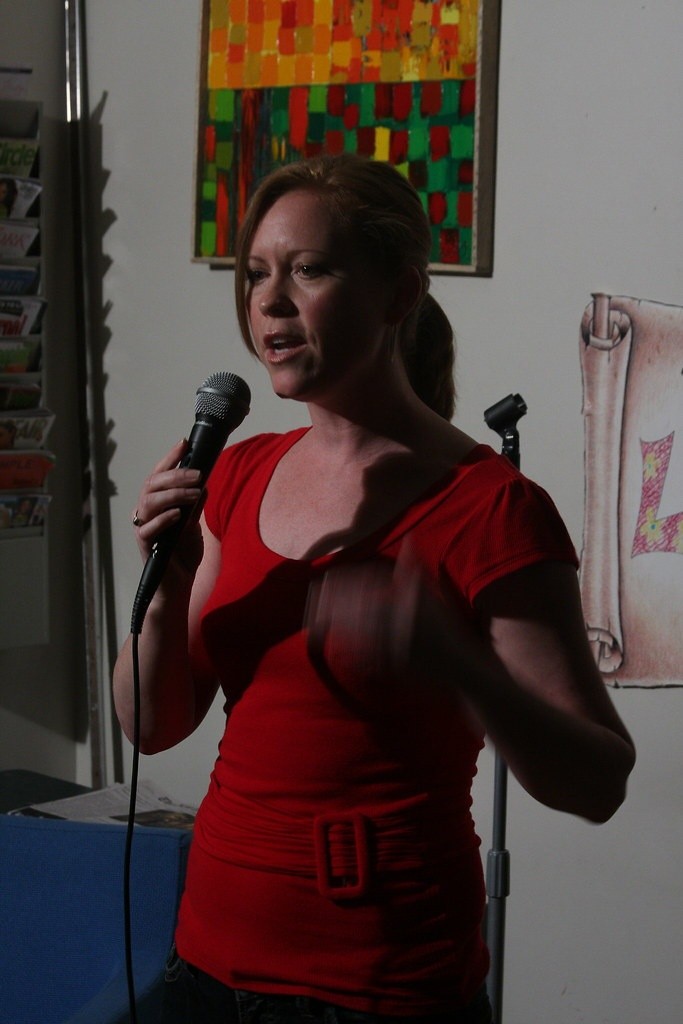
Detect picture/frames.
[189,1,500,276]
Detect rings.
[132,509,144,527]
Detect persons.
[0,420,17,448]
[0,178,18,219]
[115,156,637,1024]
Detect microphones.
[128,372,251,632]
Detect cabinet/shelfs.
[0,64,53,654]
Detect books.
[0,383,42,410]
[0,264,49,373]
[0,450,55,489]
[0,138,38,176]
[0,220,40,256]
[0,408,56,449]
[0,174,43,219]
[0,494,54,528]
